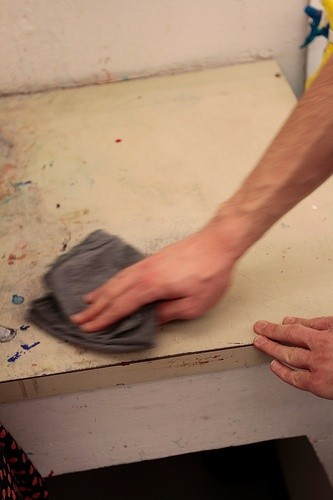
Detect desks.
[0,59,332,489]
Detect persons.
[67,47,333,402]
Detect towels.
[24,223,163,357]
